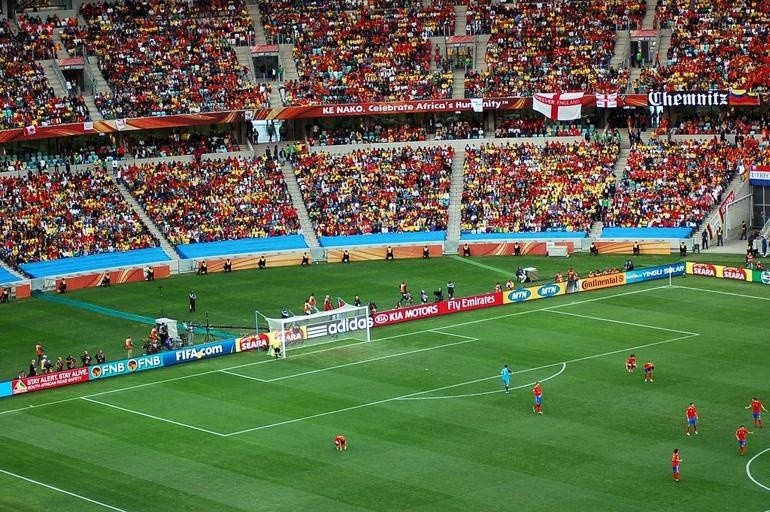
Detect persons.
[672,448,683,482]
[304,279,457,317]
[0,2,769,270]
[500,365,511,394]
[745,398,768,428]
[533,381,543,414]
[188,290,197,311]
[735,425,754,456]
[555,267,620,283]
[686,402,698,436]
[333,436,348,451]
[495,268,528,293]
[124,323,172,358]
[17,342,105,381]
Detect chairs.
[2,1,770,276]
